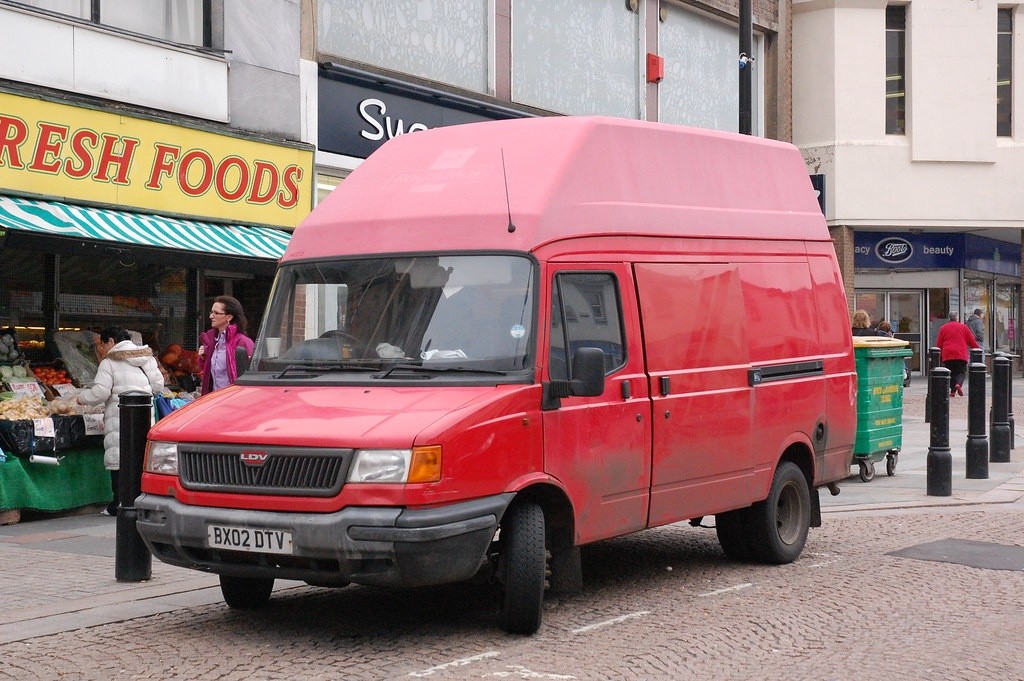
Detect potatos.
[0,396,77,420]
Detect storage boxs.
[141,330,162,352]
[0,416,72,456]
[0,327,81,402]
[59,414,104,450]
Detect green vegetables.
[0,392,15,400]
[77,341,100,366]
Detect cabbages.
[0,365,28,377]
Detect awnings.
[0,196,295,263]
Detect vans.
[132,114,860,638]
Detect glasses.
[210,311,229,315]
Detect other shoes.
[950,391,956,397]
[955,383,963,396]
[103,500,120,515]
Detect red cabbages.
[0,334,18,362]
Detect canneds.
[342,344,351,361]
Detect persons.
[851,310,876,336]
[966,309,990,378]
[198,295,255,397]
[875,321,907,379]
[76,325,164,517]
[937,311,979,396]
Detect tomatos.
[35,367,72,386]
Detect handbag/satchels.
[155,392,171,421]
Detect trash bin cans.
[903,349,913,387]
[851,335,914,482]
